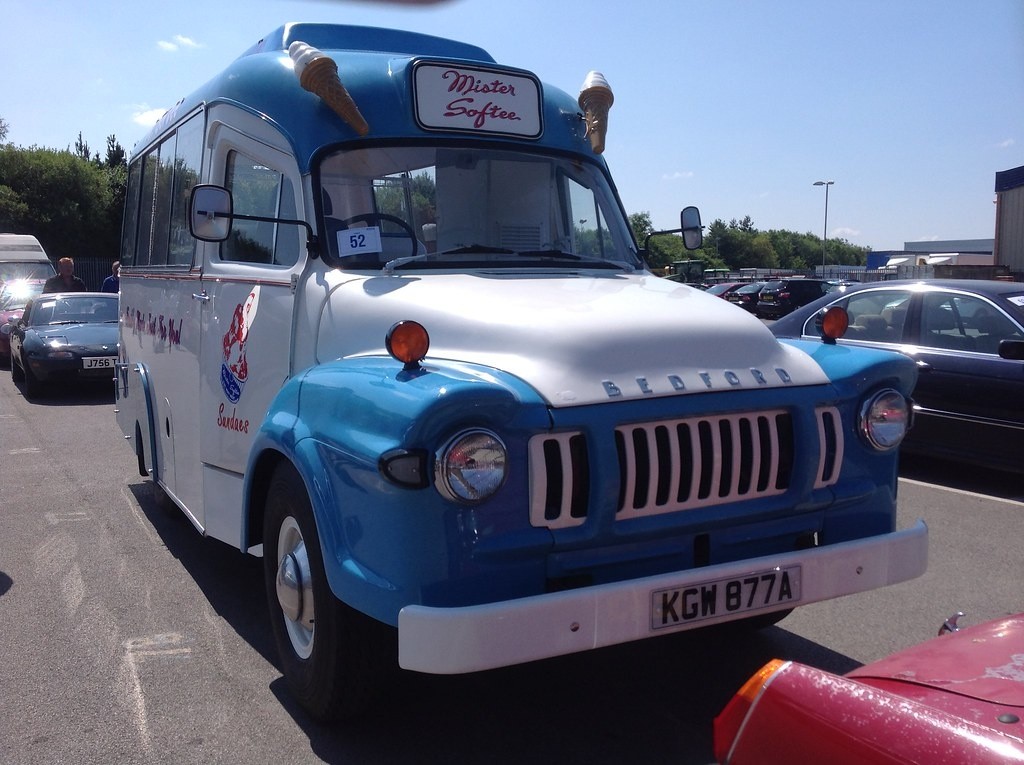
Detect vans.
[0,233,57,286]
[758,277,836,318]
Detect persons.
[42,257,87,294]
[101,261,119,293]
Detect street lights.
[811,181,834,279]
[715,237,721,259]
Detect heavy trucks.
[877,254,994,277]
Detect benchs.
[842,307,907,343]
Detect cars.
[825,280,864,301]
[0,279,48,356]
[2,293,119,397]
[711,610,1023,765]
[767,276,1023,468]
[684,281,766,309]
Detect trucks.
[116,14,930,698]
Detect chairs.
[975,316,1017,355]
[39,307,59,324]
[94,307,114,321]
[925,308,959,351]
[256,179,357,265]
